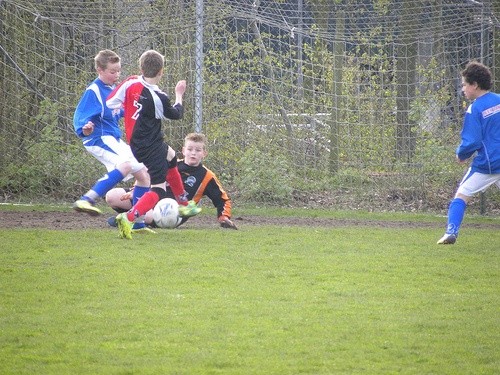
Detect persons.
[106,133,238,229]
[105,49,203,240]
[437,61,499,244]
[72,49,156,235]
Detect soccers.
[153,198,182,228]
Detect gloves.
[217,216,237,230]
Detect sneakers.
[179,199,203,217]
[108,216,117,227]
[115,212,136,240]
[71,199,103,217]
[437,233,457,244]
[130,226,156,234]
